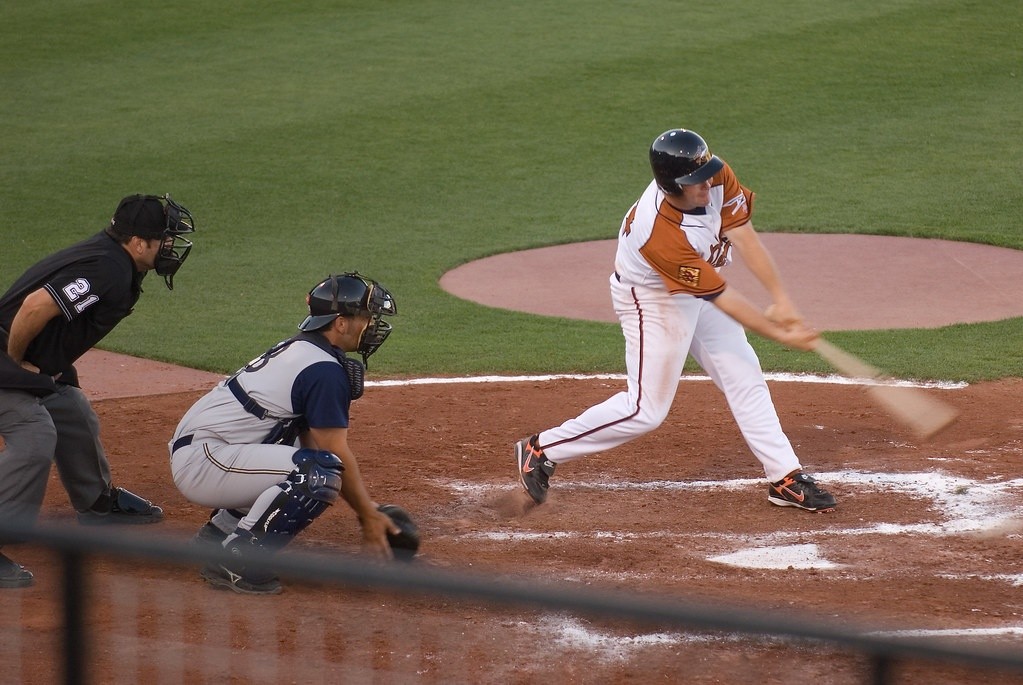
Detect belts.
[615,269,621,282]
[171,433,195,454]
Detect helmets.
[296,274,372,331]
[649,128,725,197]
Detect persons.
[0,192,196,588]
[515,127,838,513]
[168,273,402,597]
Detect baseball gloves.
[359,501,420,562]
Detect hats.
[110,192,164,236]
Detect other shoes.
[0,551,34,588]
[75,481,164,525]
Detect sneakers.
[190,520,228,552]
[200,557,283,595]
[514,433,557,505]
[768,468,838,513]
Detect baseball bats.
[768,304,963,440]
